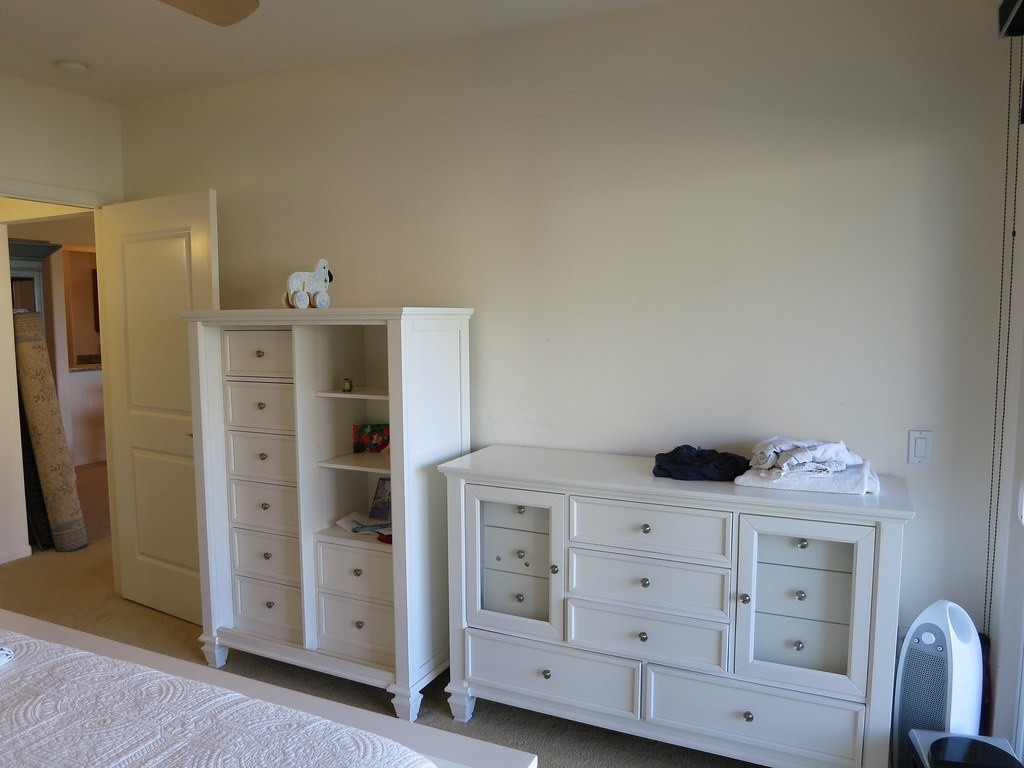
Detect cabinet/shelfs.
[439,444,914,768]
[175,307,474,721]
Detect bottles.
[342,378,352,392]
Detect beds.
[0,609,541,768]
